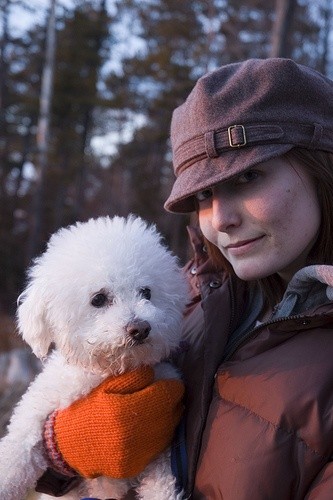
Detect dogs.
[0,213,192,499]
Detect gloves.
[42,366,187,480]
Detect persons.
[0,58,332,500]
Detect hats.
[163,57,333,215]
